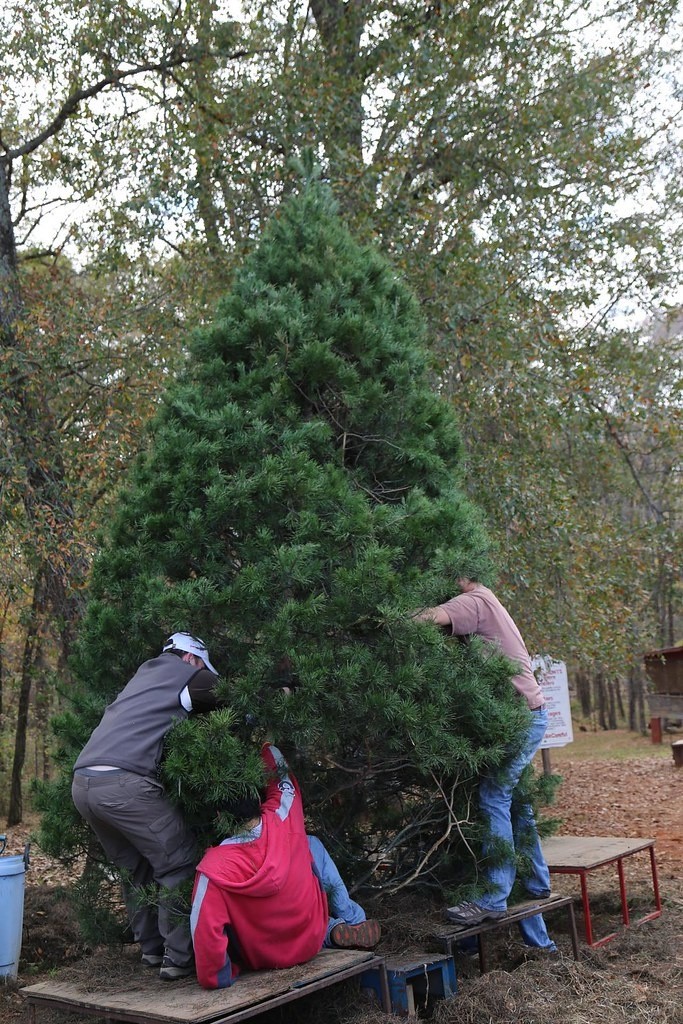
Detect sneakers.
[159,955,196,979]
[446,899,507,925]
[141,954,164,964]
[510,880,551,901]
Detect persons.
[411,566,560,954]
[73,633,382,989]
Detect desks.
[418,892,581,974]
[19,948,392,1024]
[540,835,663,950]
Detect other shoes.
[332,918,381,948]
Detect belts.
[530,703,546,712]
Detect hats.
[163,633,218,677]
